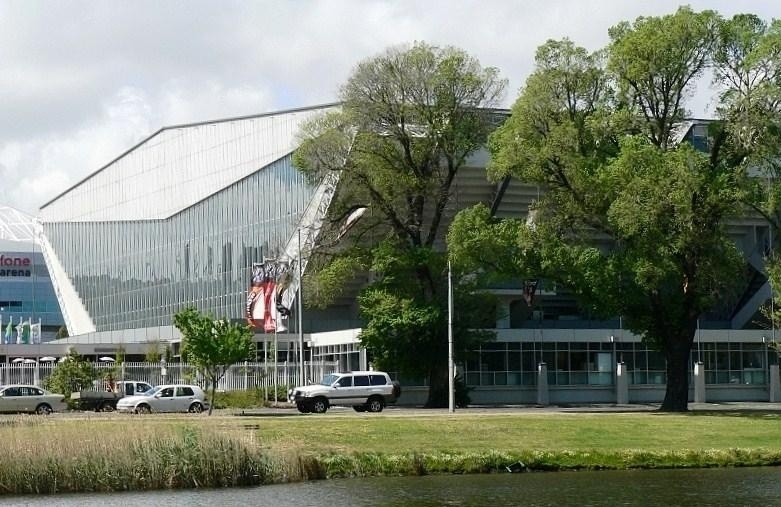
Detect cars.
[116,384,210,415]
[0,384,69,416]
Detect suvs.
[289,369,401,414]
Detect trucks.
[69,379,154,413]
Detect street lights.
[296,215,330,388]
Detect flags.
[0,315,42,345]
